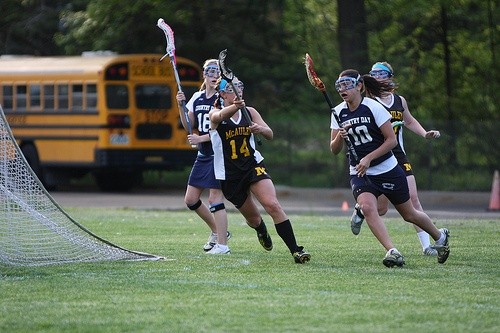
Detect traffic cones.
[486,170,499,211]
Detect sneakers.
[205,244,231,254]
[423,245,438,257]
[256,225,273,251]
[351,203,365,235]
[291,246,311,264]
[435,228,451,264]
[382,250,405,269]
[204,232,231,251]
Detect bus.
[0,51,203,191]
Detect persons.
[351,62,438,256]
[330,70,450,268]
[208,75,311,264]
[176,59,230,254]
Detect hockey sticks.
[305,52,372,186]
[218,48,263,146]
[157,18,198,149]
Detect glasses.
[223,81,244,94]
[369,70,390,80]
[335,77,358,92]
[205,65,222,77]
